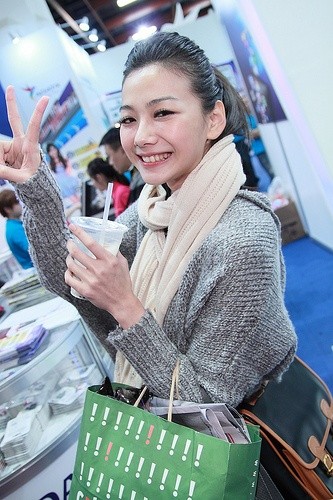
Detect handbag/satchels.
[66,357,262,499]
[236,354,333,500]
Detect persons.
[0,189,34,271]
[227,88,274,188]
[45,143,79,198]
[86,158,130,218]
[1,29,298,500]
[97,125,146,222]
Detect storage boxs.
[272,197,305,246]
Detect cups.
[69,217,128,300]
